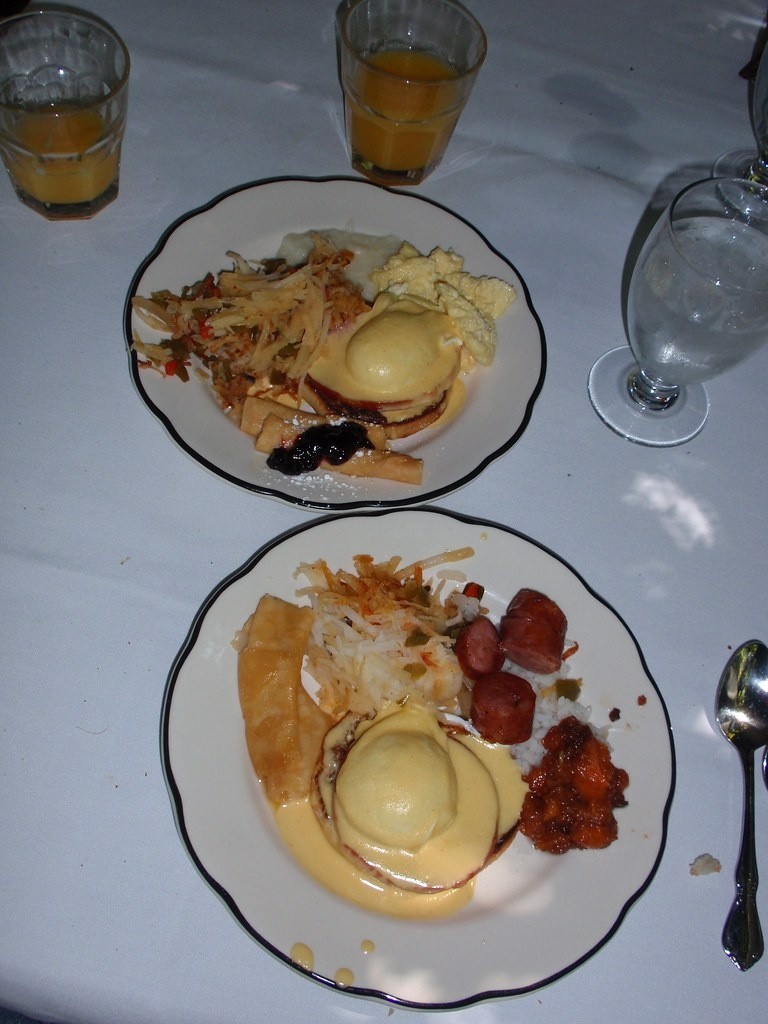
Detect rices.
[454,596,612,773]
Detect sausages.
[452,587,568,747]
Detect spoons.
[715,640,768,975]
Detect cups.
[337,0,489,189]
[0,8,137,225]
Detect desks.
[0,0,768,1023]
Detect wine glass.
[713,40,768,226]
[588,174,768,448]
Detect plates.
[123,175,549,514]
[159,509,675,1011]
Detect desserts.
[307,696,529,896]
[298,292,460,439]
